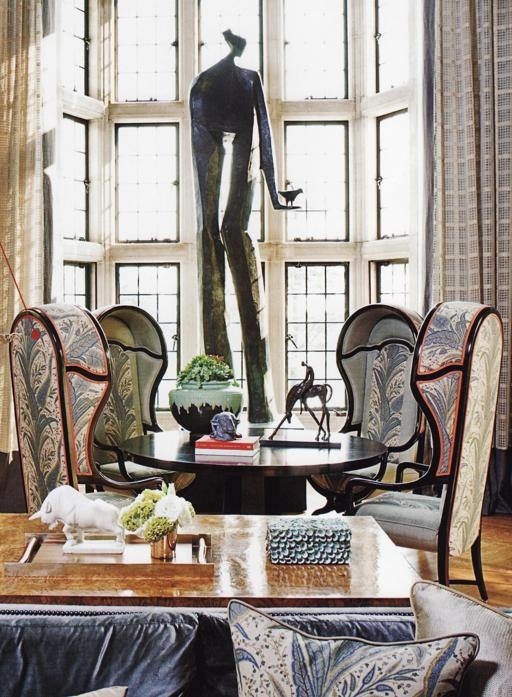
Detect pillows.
[223,599,480,697]
[0,610,203,697]
[407,578,512,697]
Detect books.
[194,435,260,456]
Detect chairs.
[305,303,431,514]
[8,304,166,515]
[90,305,197,496]
[354,301,505,601]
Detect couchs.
[0,596,512,697]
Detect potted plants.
[119,482,195,561]
[169,354,243,446]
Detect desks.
[0,511,425,607]
